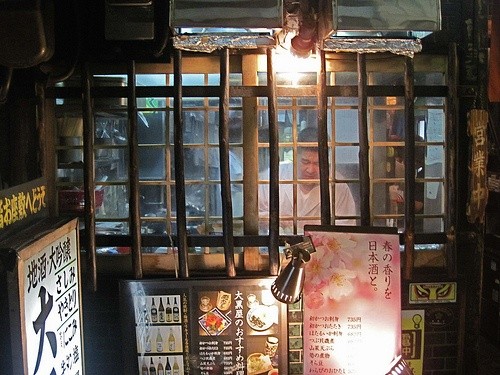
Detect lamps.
[288,20,316,58]
[271,239,311,305]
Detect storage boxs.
[59,189,105,213]
[63,77,127,106]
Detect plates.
[267,368,278,375]
[247,352,265,362]
[270,355,279,366]
[247,300,260,309]
[198,308,231,337]
[245,305,275,330]
[200,303,214,312]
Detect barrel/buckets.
[58,135,83,163]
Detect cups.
[215,295,231,311]
[247,293,256,305]
[261,290,276,306]
[199,295,210,307]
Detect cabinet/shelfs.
[55,109,128,218]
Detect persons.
[389,136,425,211]
[211,115,261,252]
[255,128,357,255]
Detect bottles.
[168,328,176,351]
[144,328,151,351]
[142,357,179,375]
[155,328,164,352]
[140,297,180,322]
